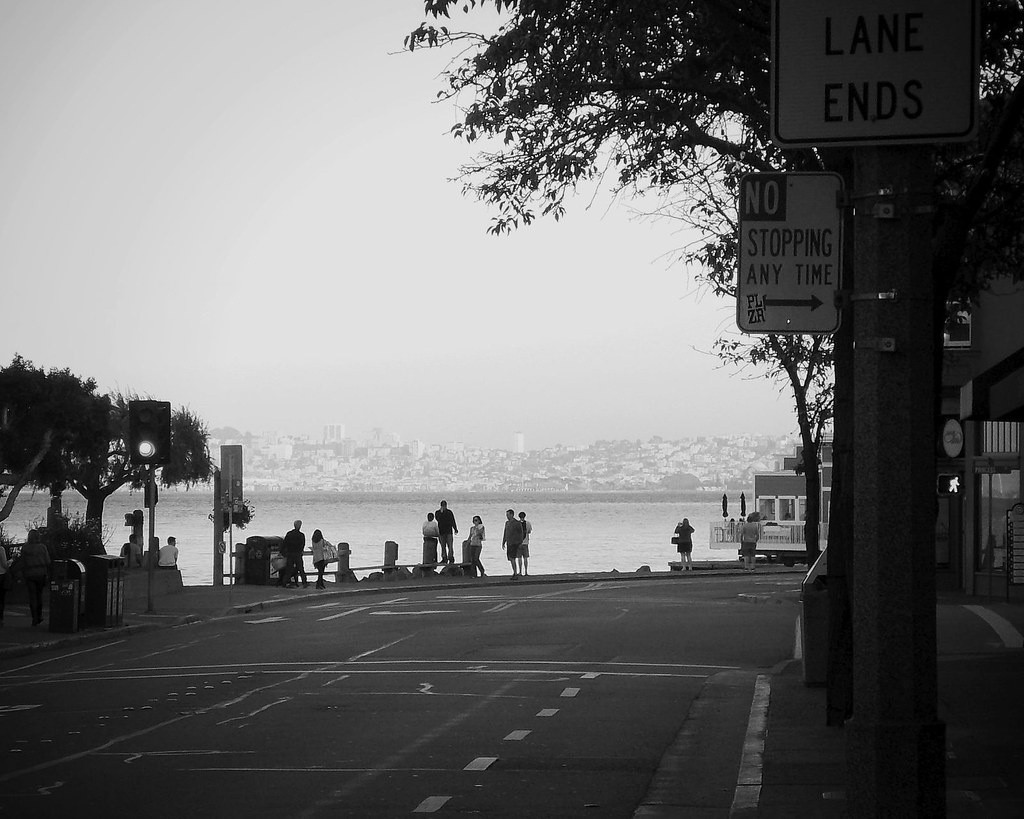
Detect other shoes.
[38,615,43,623]
[752,568,755,571]
[745,568,748,571]
[32,620,37,626]
[449,557,454,564]
[439,559,447,564]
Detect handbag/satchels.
[322,540,337,563]
[671,537,679,544]
[9,557,25,584]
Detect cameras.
[681,523,682,525]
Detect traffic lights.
[937,474,963,496]
[127,401,161,464]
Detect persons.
[434,500,459,564]
[158,536,178,570]
[726,518,746,542]
[517,512,532,576]
[21,529,52,627]
[739,515,760,557]
[280,520,310,588]
[465,516,485,578]
[120,533,142,569]
[502,509,523,579]
[311,529,330,589]
[422,512,438,538]
[674,518,694,570]
[0,546,16,626]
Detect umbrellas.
[722,493,728,521]
[740,491,747,520]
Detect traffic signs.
[735,171,846,335]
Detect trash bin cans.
[246,536,285,586]
[86,555,126,628]
[798,547,830,688]
